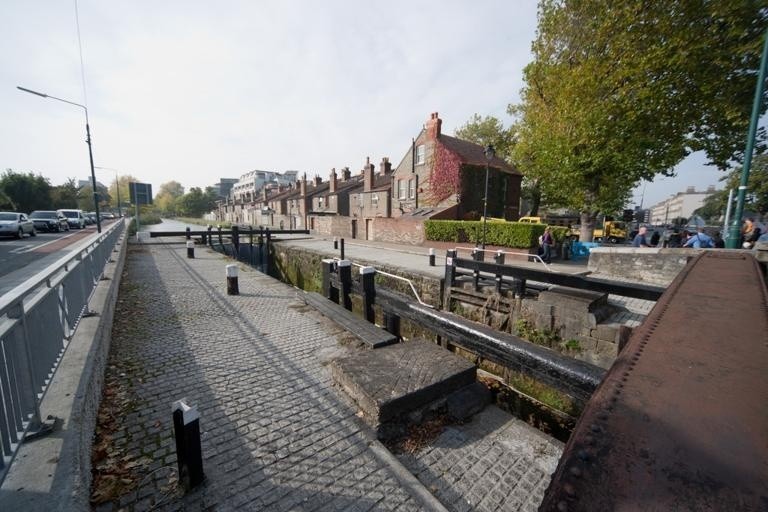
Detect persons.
[533,226,556,264]
[631,226,657,249]
[662,212,768,252]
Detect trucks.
[570,219,627,244]
[516,216,571,244]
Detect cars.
[644,222,721,241]
[0,207,115,241]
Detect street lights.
[16,86,101,233]
[94,166,121,218]
[480,140,496,250]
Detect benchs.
[296,292,397,348]
